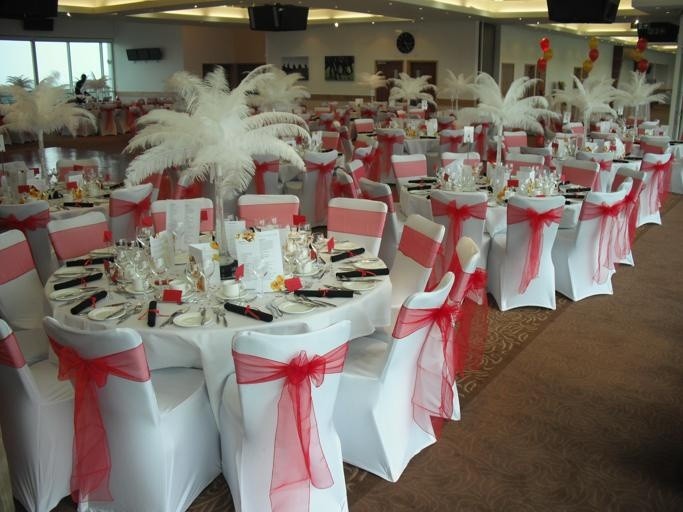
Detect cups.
[295,257,312,274]
[82,168,106,197]
[221,280,244,298]
[169,280,190,295]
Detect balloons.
[534,36,650,76]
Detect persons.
[75,73,91,102]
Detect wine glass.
[109,224,167,304]
[437,161,563,197]
[184,259,214,302]
[252,259,270,298]
[253,215,323,287]
[45,173,61,212]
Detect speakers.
[636,21,679,42]
[248,4,308,31]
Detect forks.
[117,305,143,328]
[212,306,230,328]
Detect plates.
[289,266,320,277]
[215,291,256,301]
[277,300,317,316]
[353,262,387,270]
[53,267,86,279]
[180,292,195,300]
[340,280,378,292]
[172,312,212,328]
[86,306,127,322]
[46,288,84,302]
[334,242,359,252]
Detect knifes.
[104,307,126,320]
[199,309,207,326]
